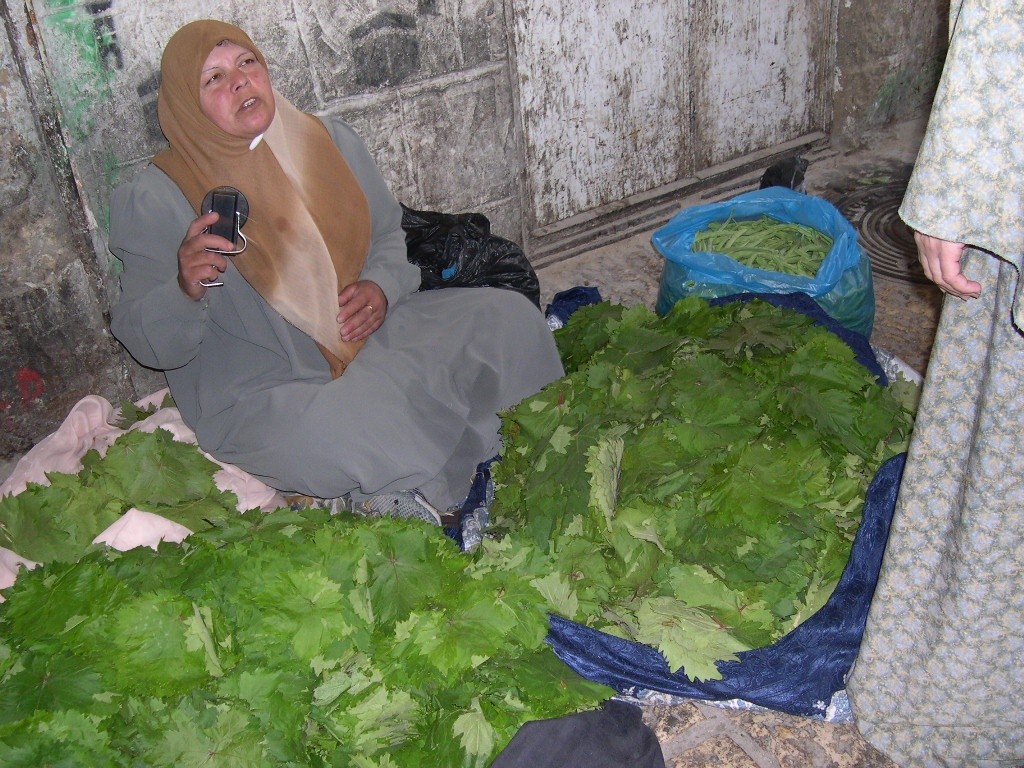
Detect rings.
[366,305,374,314]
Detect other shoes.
[354,489,443,527]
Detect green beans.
[695,211,835,282]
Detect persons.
[848,0,1023,768]
[107,20,569,539]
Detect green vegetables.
[0,391,619,768]
[492,296,919,684]
